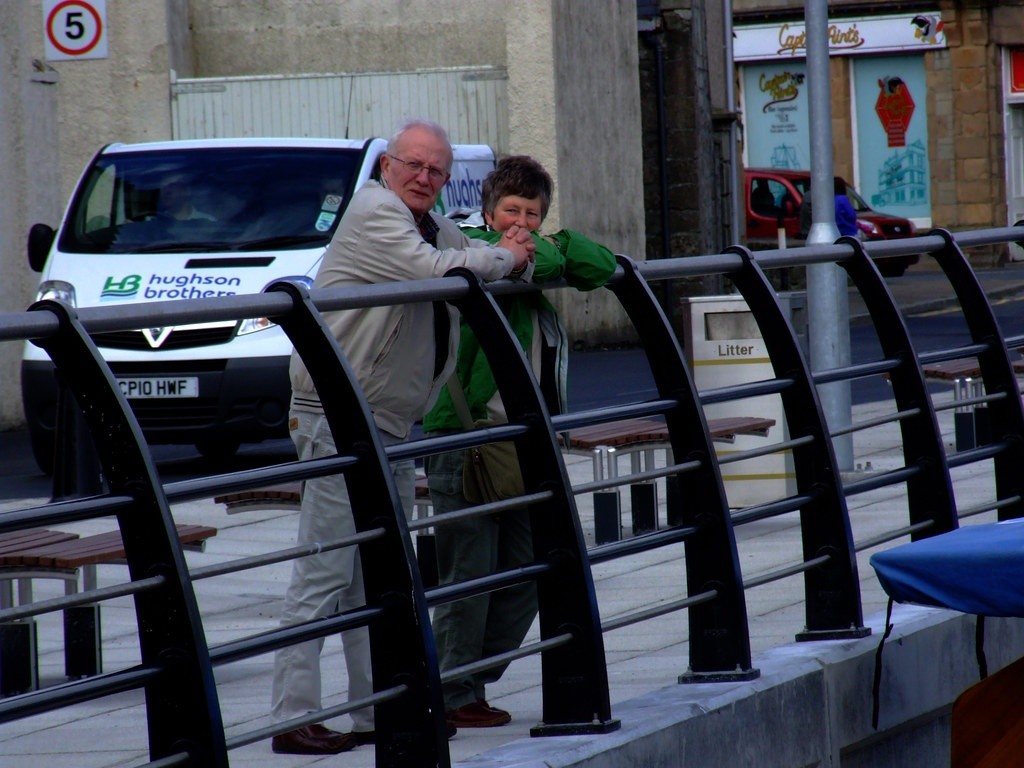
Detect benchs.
[555,417,777,546]
[885,357,1024,452]
[1,523,217,698]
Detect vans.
[23,138,498,491]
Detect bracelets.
[512,262,527,274]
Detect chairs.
[111,165,328,249]
[751,186,803,216]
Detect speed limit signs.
[38,0,110,62]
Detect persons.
[834,176,858,237]
[270,120,535,756]
[789,178,812,290]
[751,179,775,216]
[425,155,617,729]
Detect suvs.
[742,167,918,278]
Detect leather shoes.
[272,723,356,755]
[351,725,457,746]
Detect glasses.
[386,154,448,180]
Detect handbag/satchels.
[461,418,527,509]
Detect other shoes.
[445,699,511,727]
